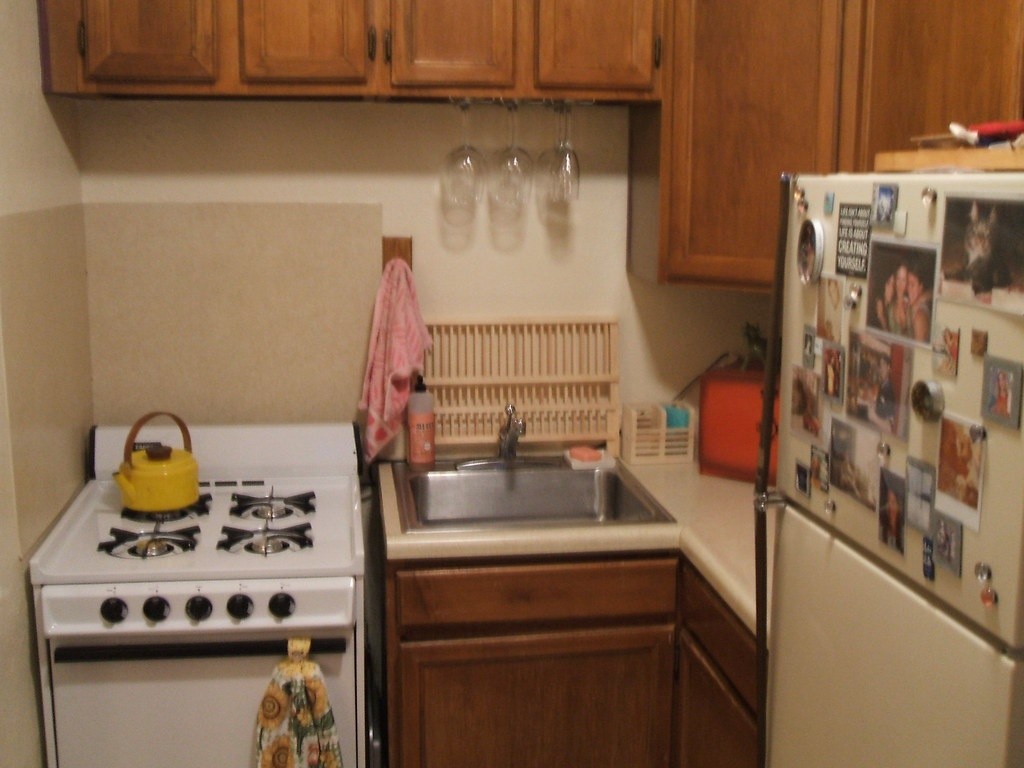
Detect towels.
[357,256,436,468]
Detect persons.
[940,328,958,378]
[875,353,897,419]
[868,265,932,346]
[877,487,904,552]
[994,373,1010,419]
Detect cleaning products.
[407,375,436,471]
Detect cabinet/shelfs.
[377,0,667,102]
[38,0,383,97]
[376,558,766,768]
[623,0,1024,290]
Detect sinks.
[390,456,678,536]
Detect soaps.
[569,445,604,464]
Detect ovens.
[46,637,356,768]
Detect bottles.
[407,374,435,470]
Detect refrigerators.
[755,173,1024,768]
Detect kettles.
[112,412,200,513]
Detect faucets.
[497,404,528,461]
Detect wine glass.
[439,95,486,201]
[488,98,532,201]
[537,100,581,203]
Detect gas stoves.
[29,421,365,639]
